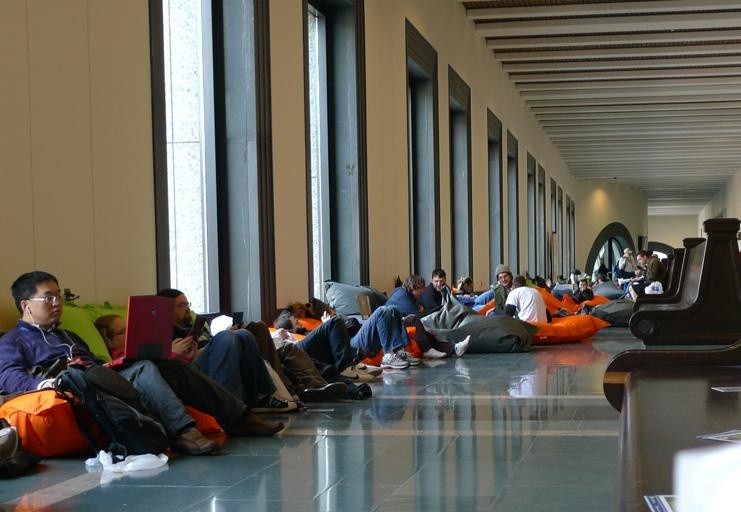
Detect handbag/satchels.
[344,317,361,337]
[53,364,169,465]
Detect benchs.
[605,216,741,511]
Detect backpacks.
[0,418,42,479]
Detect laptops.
[102,295,176,369]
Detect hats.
[496,264,512,281]
[623,248,632,254]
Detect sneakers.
[423,348,447,359]
[248,393,305,414]
[454,334,473,358]
[327,362,383,384]
[174,425,218,456]
[302,381,348,403]
[380,351,421,369]
[232,414,285,439]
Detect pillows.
[422,277,633,362]
[0,297,422,457]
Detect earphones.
[25,306,31,313]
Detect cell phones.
[233,312,243,325]
[184,328,202,340]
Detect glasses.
[176,303,191,308]
[24,294,66,304]
